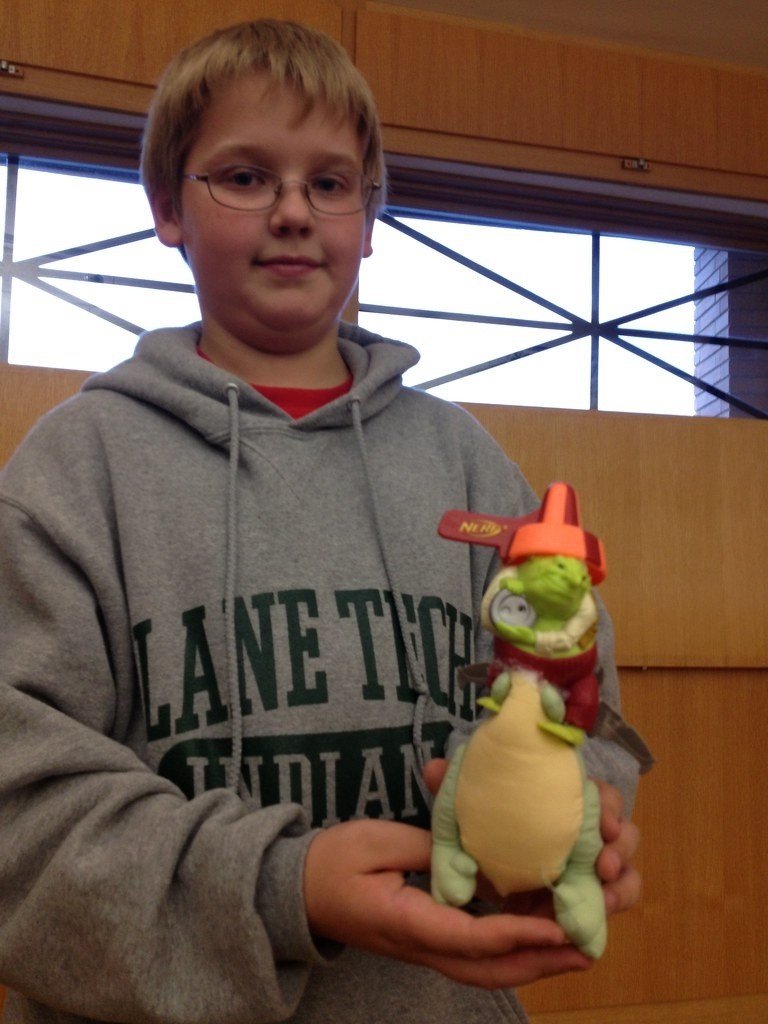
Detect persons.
[0,21,642,1024]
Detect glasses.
[179,163,381,215]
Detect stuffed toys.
[430,482,607,964]
